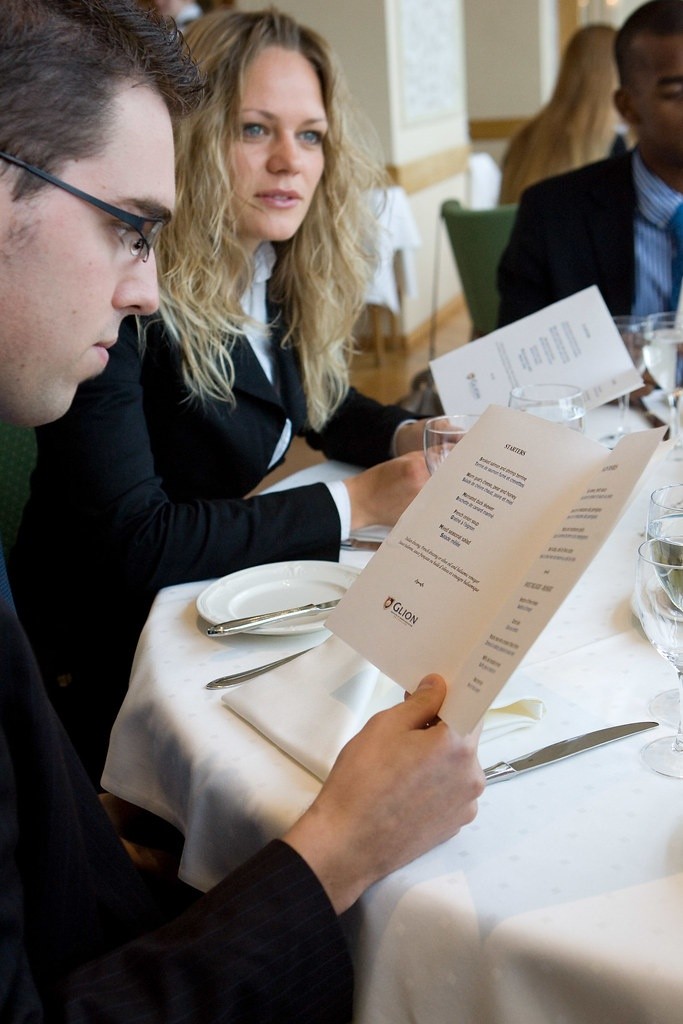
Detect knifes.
[342,539,383,551]
[482,721,660,786]
[205,598,341,638]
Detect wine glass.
[646,484,682,729]
[423,414,483,477]
[636,535,683,781]
[642,311,683,464]
[508,384,585,437]
[596,315,647,451]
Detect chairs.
[443,197,518,345]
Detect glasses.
[0,153,166,263]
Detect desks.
[355,185,424,370]
[99,403,683,1024]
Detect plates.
[196,560,363,634]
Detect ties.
[0,543,19,623]
[665,202,683,312]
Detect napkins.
[220,633,546,784]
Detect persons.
[495,0,683,441]
[0,1,484,1024]
